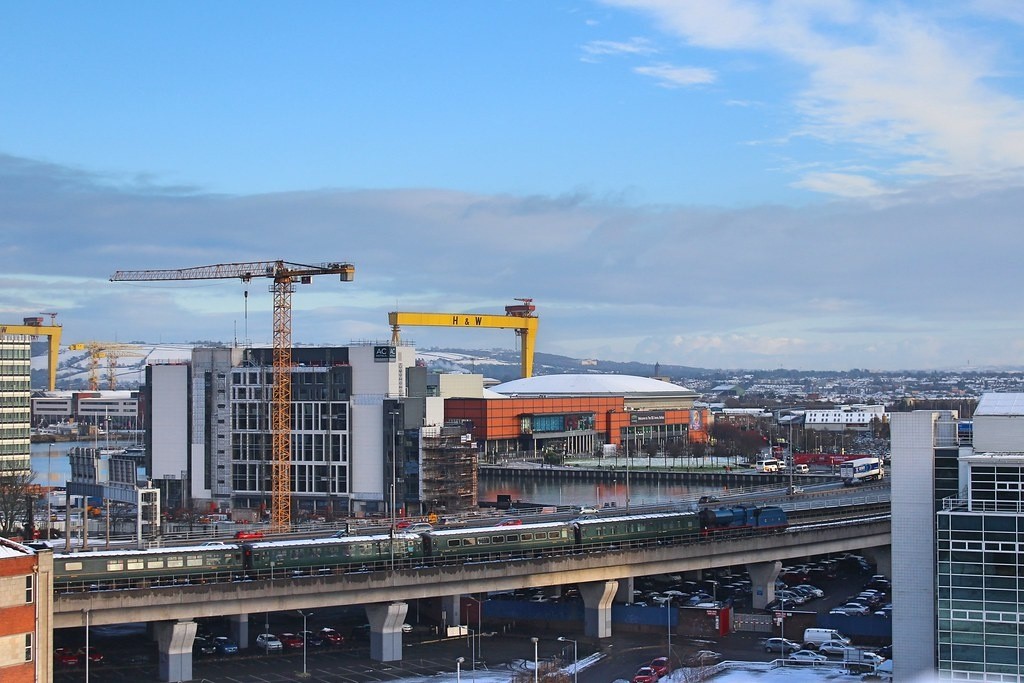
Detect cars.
[755,446,810,474]
[493,518,522,527]
[698,494,720,505]
[573,505,600,515]
[786,485,805,496]
[388,515,469,535]
[200,541,224,546]
[330,529,357,538]
[570,513,598,521]
[53,554,893,683]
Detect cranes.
[0,311,64,391]
[108,256,357,533]
[387,297,540,378]
[68,341,148,391]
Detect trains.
[52,500,790,593]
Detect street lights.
[814,431,822,454]
[783,401,796,495]
[457,624,474,683]
[557,636,578,683]
[297,610,314,674]
[781,584,789,667]
[82,608,90,683]
[614,479,618,509]
[667,592,675,680]
[389,412,399,529]
[837,434,843,454]
[456,656,465,683]
[466,603,472,648]
[800,429,807,454]
[469,597,481,658]
[531,637,539,683]
[47,444,55,542]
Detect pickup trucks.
[233,530,265,543]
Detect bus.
[840,456,884,488]
